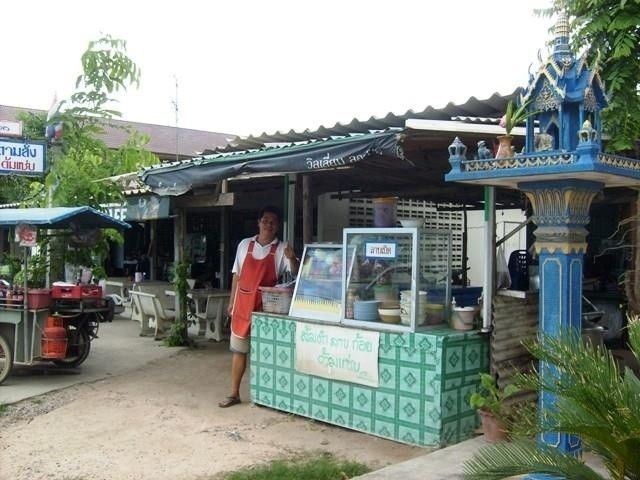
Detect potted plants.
[26,256,50,309]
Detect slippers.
[219,395,241,407]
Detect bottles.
[6,283,24,310]
[345,288,355,318]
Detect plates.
[352,300,381,321]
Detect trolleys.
[0,294,111,384]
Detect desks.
[106,280,168,307]
[166,289,232,337]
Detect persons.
[217,203,300,408]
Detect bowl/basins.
[376,306,401,323]
[400,288,428,327]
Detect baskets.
[258,286,293,314]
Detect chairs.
[128,291,176,341]
[196,294,231,343]
